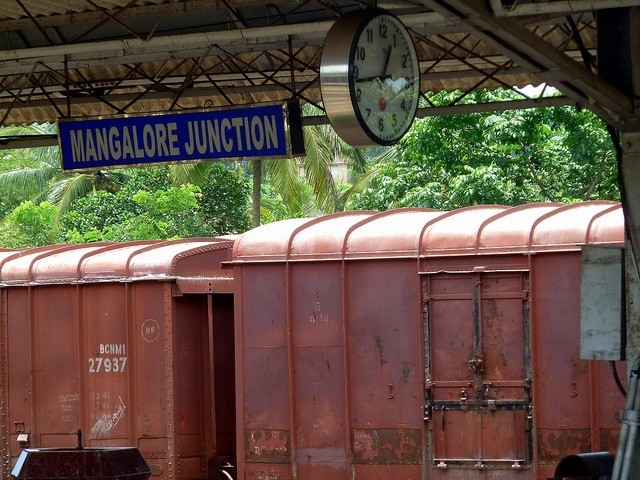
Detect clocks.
[318,6,422,147]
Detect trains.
[1,202,638,479]
